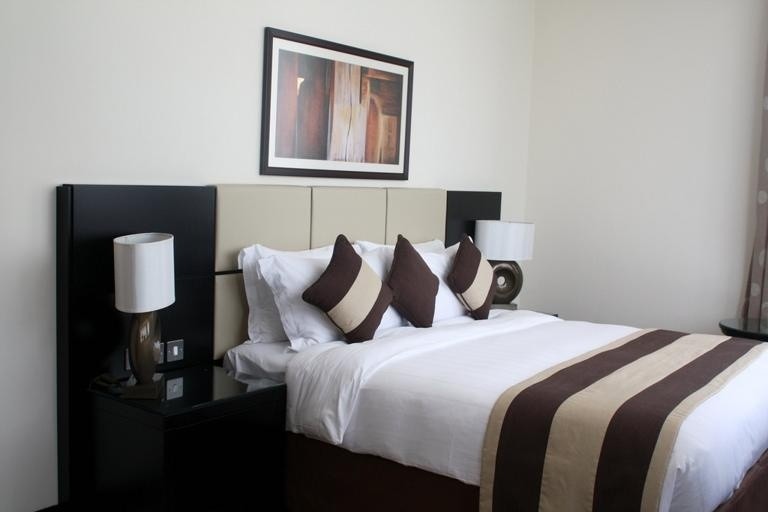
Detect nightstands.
[91,365,286,512]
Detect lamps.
[114,233,178,400]
[475,219,535,310]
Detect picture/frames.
[260,26,414,180]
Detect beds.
[56,183,768,512]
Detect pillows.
[237,232,498,353]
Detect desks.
[718,318,768,342]
